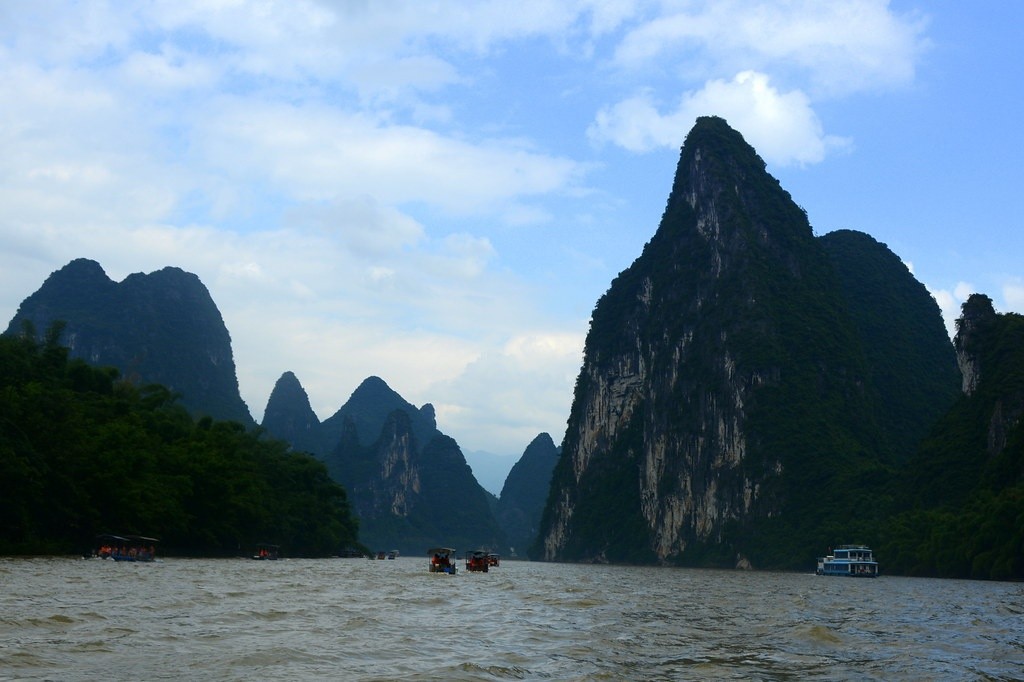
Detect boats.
[253,555,273,561]
[99,550,154,562]
[815,545,880,579]
[428,547,456,574]
[487,553,500,567]
[377,553,396,560]
[465,550,489,572]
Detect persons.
[432,553,449,566]
[99,542,156,561]
[469,555,497,566]
